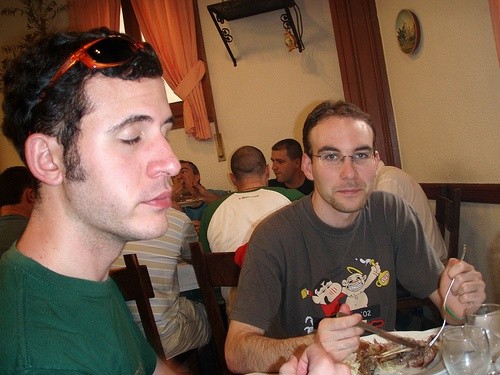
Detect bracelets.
[444,306,466,320]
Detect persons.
[110,137,315,375]
[225,101,486,373]
[374,161,448,262]
[0,166,38,254]
[0,26,353,375]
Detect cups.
[464,304,500,375]
[442,326,491,375]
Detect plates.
[360,331,447,375]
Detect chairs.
[396,184,462,328]
[189,242,241,375]
[109,254,167,360]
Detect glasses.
[49,35,163,87]
[312,151,370,165]
